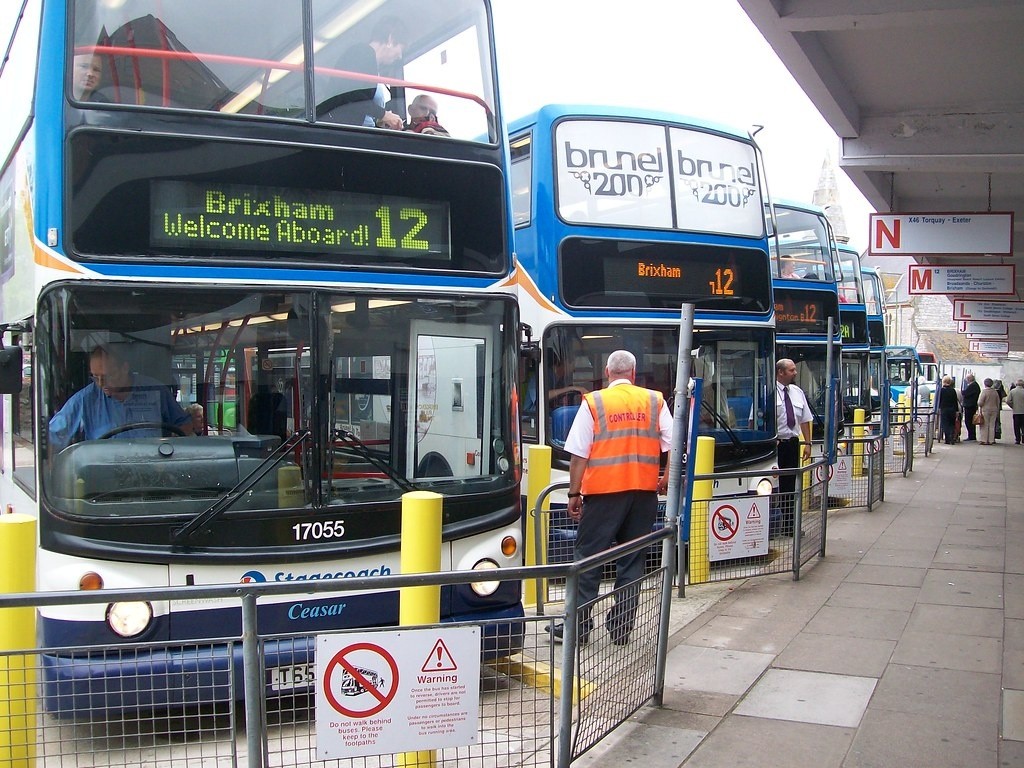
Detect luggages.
[953,412,961,442]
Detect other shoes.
[979,441,992,445]
[1015,441,1020,444]
[545,624,588,643]
[944,441,954,444]
[964,438,972,441]
[611,633,628,644]
[786,531,805,537]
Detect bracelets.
[568,492,581,498]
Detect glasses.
[90,368,119,383]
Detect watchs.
[806,442,812,446]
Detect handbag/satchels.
[972,406,985,425]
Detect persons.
[776,255,801,279]
[73,42,110,103]
[247,369,287,452]
[1006,378,1024,444]
[977,378,1004,445]
[186,403,214,436]
[1010,383,1016,391]
[904,375,963,445]
[748,359,814,537]
[402,94,439,133]
[522,353,590,422]
[962,374,980,441]
[49,343,193,462]
[545,350,674,645]
[316,15,415,130]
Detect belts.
[778,438,796,442]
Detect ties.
[783,387,796,430]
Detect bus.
[763,196,845,455]
[886,346,924,414]
[830,265,889,410]
[917,352,937,403]
[244,105,781,558]
[0,0,538,719]
[170,348,237,428]
[808,243,869,434]
